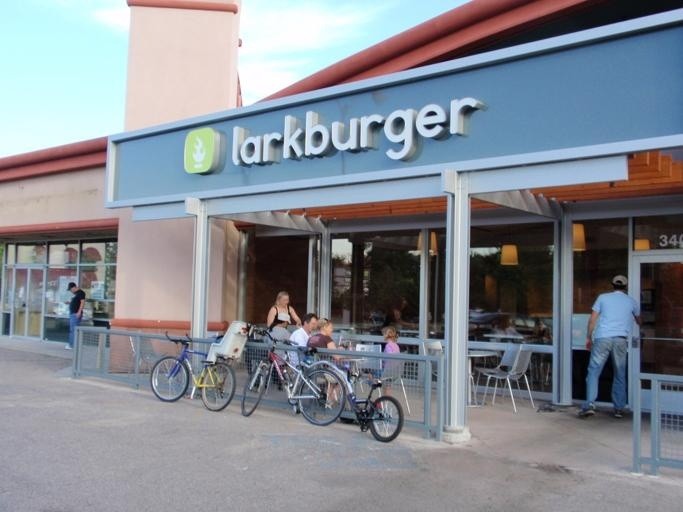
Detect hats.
[277,312,291,325]
[68,283,74,290]
[612,275,628,286]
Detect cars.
[469,312,553,372]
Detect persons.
[63,282,86,350]
[488,313,517,383]
[380,295,418,351]
[266,290,354,410]
[367,326,401,419]
[524,319,553,385]
[576,274,645,419]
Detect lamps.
[634,239,650,251]
[500,244,518,265]
[572,224,587,251]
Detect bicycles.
[150,330,236,411]
[335,373,404,443]
[240,323,347,426]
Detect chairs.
[356,336,535,416]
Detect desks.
[418,232,437,255]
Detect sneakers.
[579,409,595,416]
[614,408,623,417]
